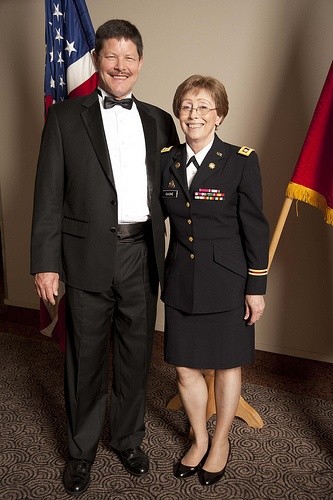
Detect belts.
[117,221,152,240]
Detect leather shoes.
[176,433,210,477]
[110,446,149,476]
[63,456,91,496]
[199,438,231,485]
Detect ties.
[97,89,133,110]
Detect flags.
[39,0,100,354]
[287,60,333,225]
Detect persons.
[159,74,270,485]
[30,19,181,496]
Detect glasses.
[177,105,220,115]
